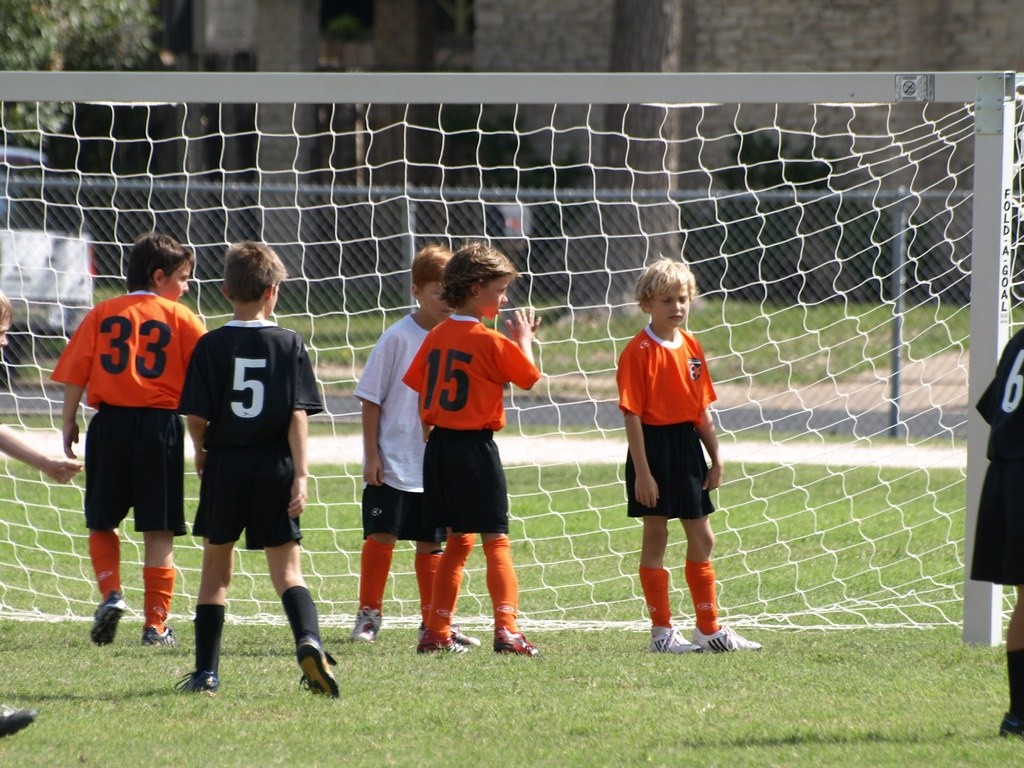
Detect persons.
[349,245,483,646]
[0,289,83,736]
[970,327,1024,739]
[50,234,207,642]
[176,242,341,699]
[616,259,761,654]
[402,242,541,654]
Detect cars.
[1,146,98,389]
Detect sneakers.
[176,672,219,698]
[692,624,762,653]
[493,627,540,656]
[296,636,338,698]
[352,606,382,645]
[417,629,469,654]
[140,624,176,652]
[90,591,126,646]
[648,626,702,655]
[418,623,482,651]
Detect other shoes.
[0,704,35,740]
[999,713,1024,738]
